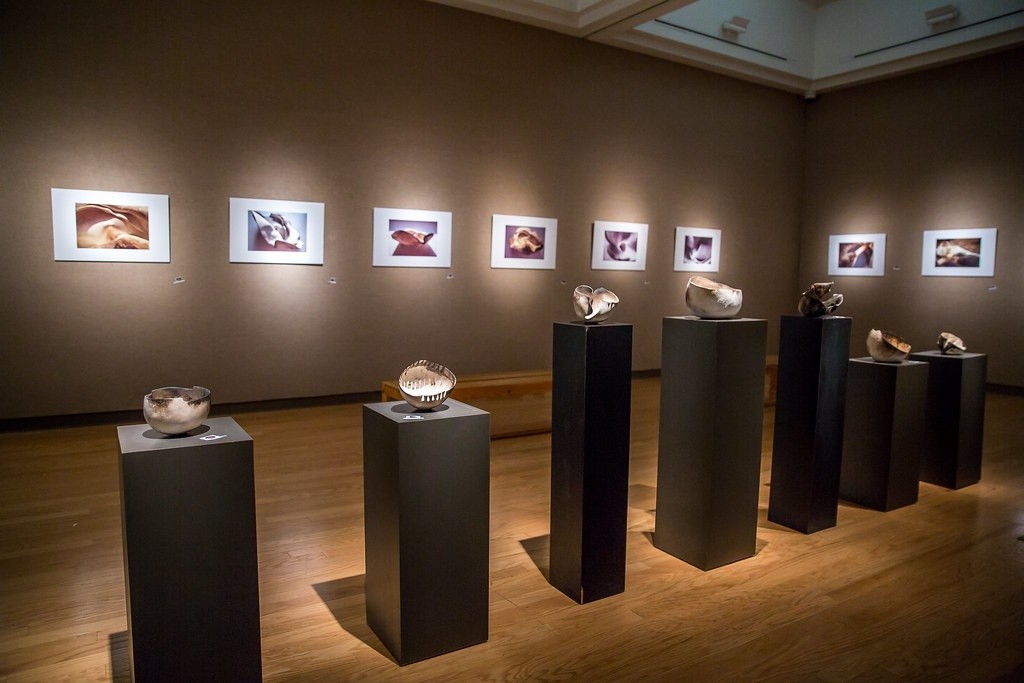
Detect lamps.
[722,14,750,34]
[924,4,960,26]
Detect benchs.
[764,355,780,408]
[382,370,552,440]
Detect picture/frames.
[828,233,886,276]
[372,208,452,268]
[591,220,649,271]
[921,227,997,277]
[674,227,722,273]
[489,215,559,270]
[51,188,171,263]
[229,197,323,265]
